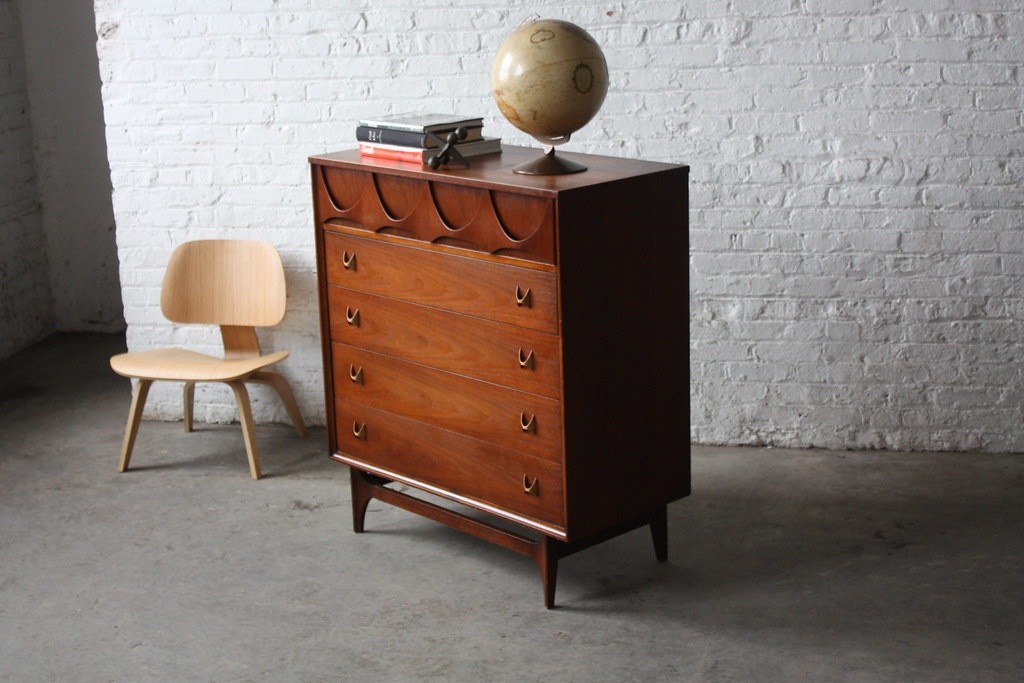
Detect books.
[356,126,485,148]
[358,135,503,165]
[360,110,484,132]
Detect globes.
[492,14,609,175]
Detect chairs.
[109,237,310,480]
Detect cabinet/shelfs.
[306,141,693,609]
